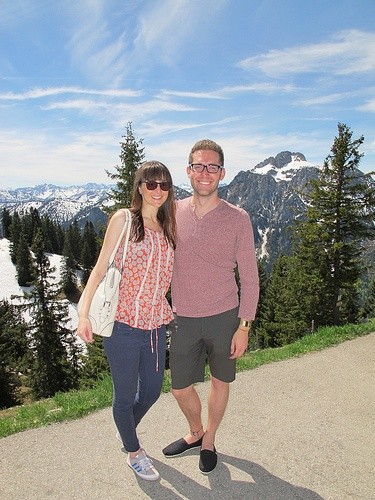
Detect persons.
[160,139,261,476]
[77,160,177,481]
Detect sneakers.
[127,448,159,480]
[116,431,124,443]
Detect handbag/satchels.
[78,208,132,338]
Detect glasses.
[140,180,172,191]
[190,164,223,174]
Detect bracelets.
[239,320,252,329]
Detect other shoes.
[162,436,205,457]
[199,444,218,474]
